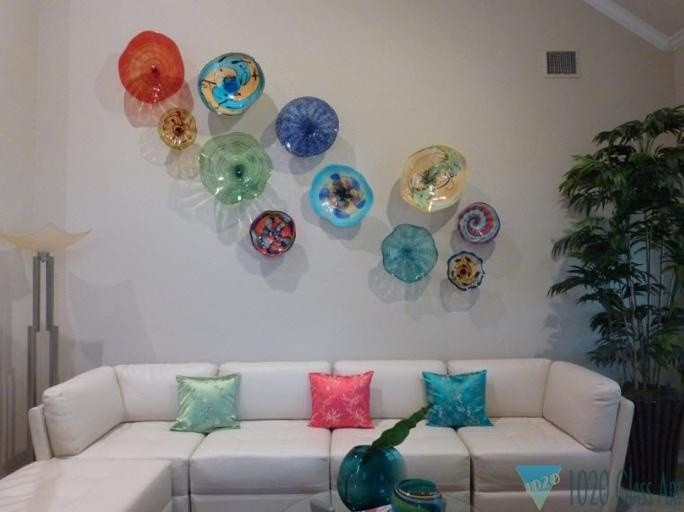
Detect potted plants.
[546,104,684,497]
[335,401,436,511]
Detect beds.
[286,490,683,511]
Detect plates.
[247,210,295,256]
[308,163,373,228]
[117,30,185,104]
[156,108,198,152]
[400,145,469,215]
[196,52,265,118]
[275,96,339,157]
[379,220,439,284]
[197,132,270,206]
[443,199,499,293]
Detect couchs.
[0,358,635,512]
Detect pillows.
[421,370,495,428]
[308,369,376,431]
[170,374,242,434]
